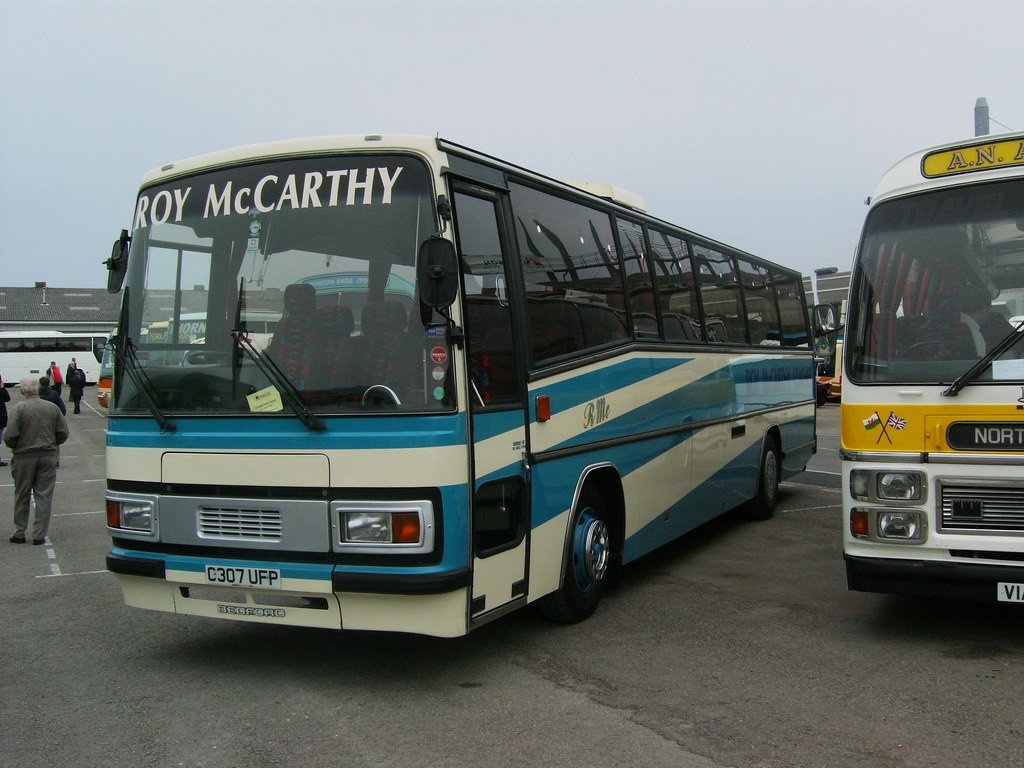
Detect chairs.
[891,312,1024,361]
[264,283,803,409]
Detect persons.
[3,377,70,546]
[0,375,11,466]
[38,376,66,416]
[46,361,64,395]
[67,357,86,414]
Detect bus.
[811,126,1024,619]
[101,131,820,643]
[0,330,111,389]
[93,309,282,408]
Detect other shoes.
[33,538,46,545]
[0,461,9,466]
[9,535,26,543]
